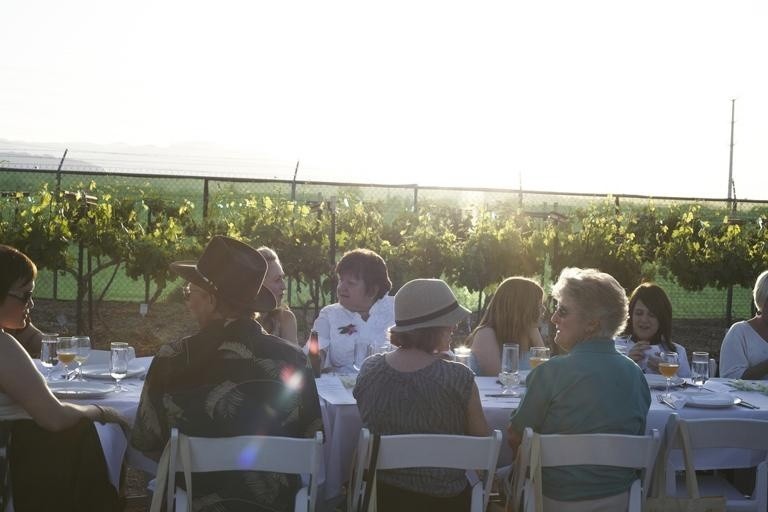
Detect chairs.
[507,426,660,512]
[165,426,324,512]
[0,419,97,511]
[653,414,768,512]
[344,427,503,512]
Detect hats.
[169,233,278,314]
[386,277,473,334]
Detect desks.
[30,358,326,511]
[315,373,767,512]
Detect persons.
[0,244,128,512]
[302,248,395,373]
[119,236,325,512]
[4,317,43,359]
[463,278,546,377]
[498,265,652,512]
[719,271,767,498]
[352,279,489,512]
[613,282,693,378]
[254,245,298,346]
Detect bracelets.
[90,403,104,412]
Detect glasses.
[556,306,586,318]
[182,283,201,297]
[7,288,32,304]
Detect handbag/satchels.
[640,419,728,512]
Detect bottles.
[307,330,323,381]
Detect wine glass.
[41,332,145,398]
[451,342,552,399]
[642,351,742,409]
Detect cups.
[351,336,369,373]
